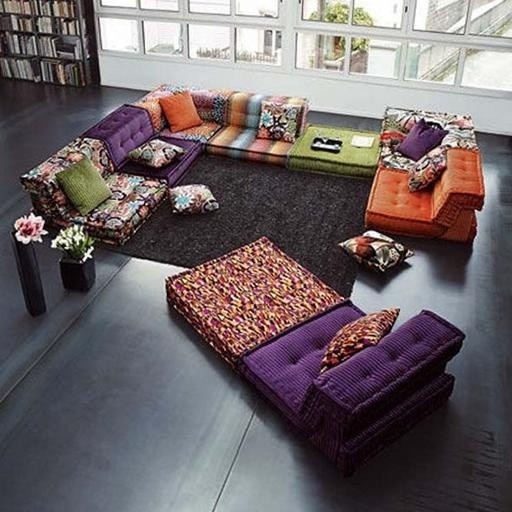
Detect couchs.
[205,90,309,166]
[20,84,234,247]
[364,107,484,245]
[287,123,381,180]
[166,236,465,478]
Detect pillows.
[129,139,183,169]
[396,119,450,162]
[256,101,301,144]
[157,91,202,133]
[338,231,415,273]
[319,308,400,374]
[54,155,112,217]
[407,147,448,193]
[168,185,220,215]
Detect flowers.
[12,214,48,243]
[49,224,94,265]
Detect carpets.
[94,151,374,298]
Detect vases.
[9,244,47,319]
[60,261,96,293]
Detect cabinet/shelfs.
[0,0,101,89]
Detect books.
[0,0,32,16]
[0,12,33,33]
[36,34,90,61]
[0,31,38,57]
[40,58,86,87]
[33,0,75,18]
[36,17,87,36]
[0,56,42,83]
